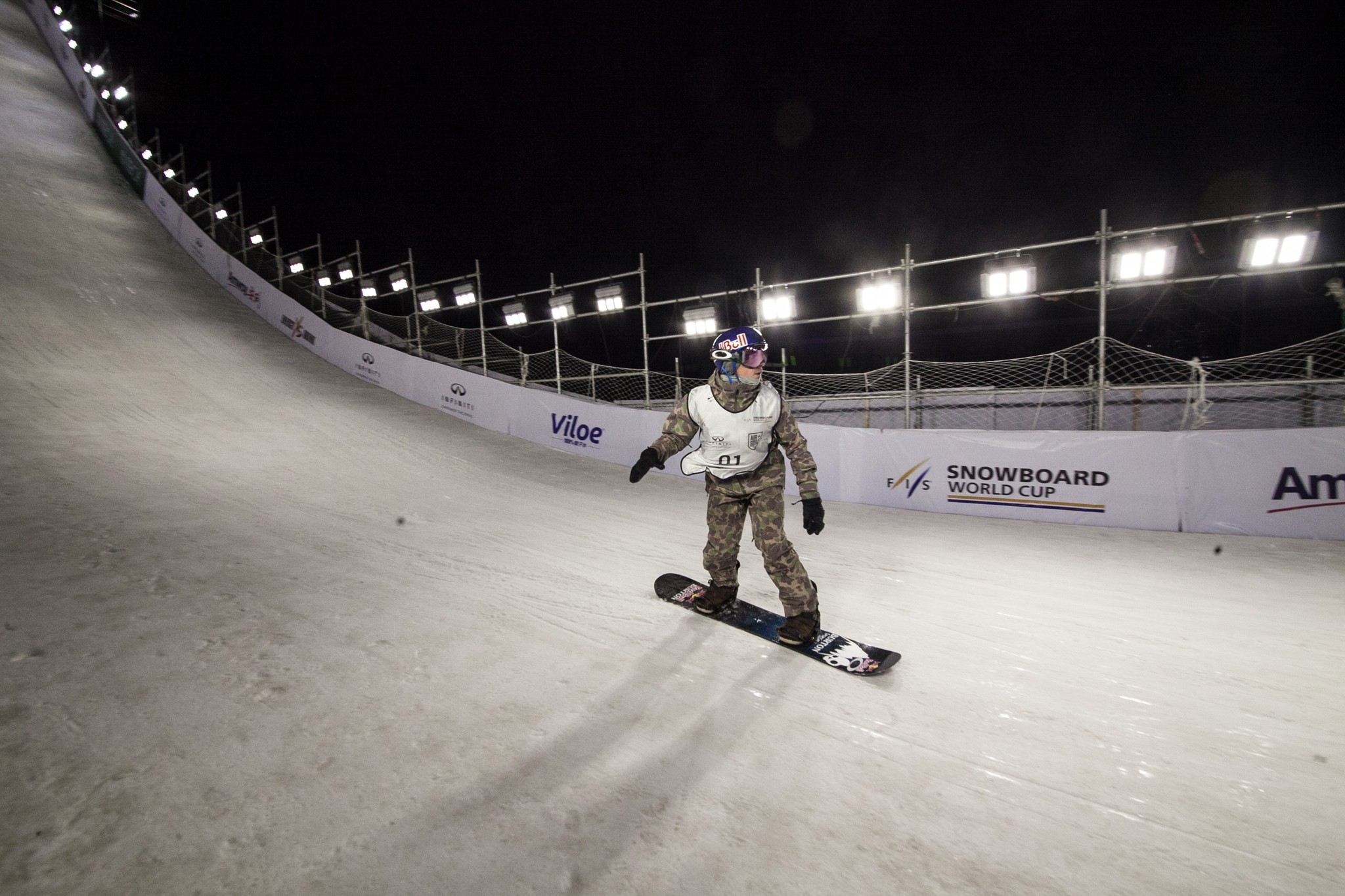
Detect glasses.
[740,345,767,368]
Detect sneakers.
[695,560,740,613]
[776,580,821,644]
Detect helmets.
[712,326,764,371]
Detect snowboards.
[657,574,902,675]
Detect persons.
[629,326,823,646]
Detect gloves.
[792,497,825,535]
[629,447,665,483]
